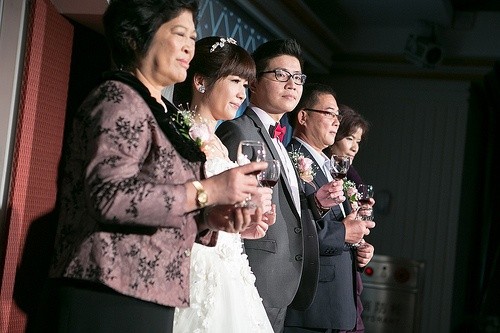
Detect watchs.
[192,181,207,208]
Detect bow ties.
[272,122,286,141]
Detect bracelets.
[203,212,217,231]
[314,196,330,211]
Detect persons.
[27,0,263,333]
[216,39,345,333]
[287,83,375,333]
[322,102,374,333]
[171,37,276,333]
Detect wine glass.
[321,154,351,203]
[353,206,374,246]
[231,140,262,208]
[256,159,280,215]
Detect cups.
[357,184,374,206]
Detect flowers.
[174,104,213,149]
[290,147,316,183]
[343,179,372,242]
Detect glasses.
[262,69,306,85]
[304,108,342,122]
